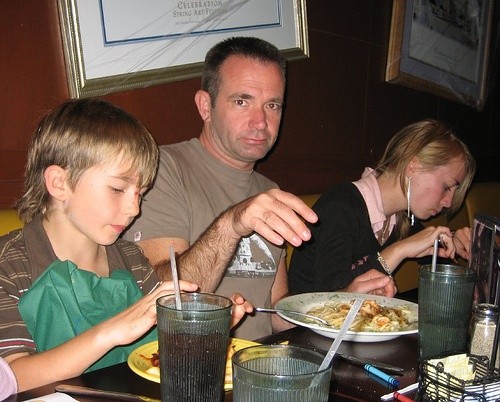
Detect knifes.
[336,353,402,372]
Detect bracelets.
[377,250,392,276]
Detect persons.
[0,97,256,393]
[288,117,477,296]
[122,35,319,341]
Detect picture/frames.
[385,0,495,112]
[55,0,309,100]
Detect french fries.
[147,344,251,382]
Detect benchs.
[0,174,499,296]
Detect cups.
[231,343,334,402]
[155,292,233,402]
[417,262,477,361]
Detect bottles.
[468,302,500,367]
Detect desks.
[2,287,499,402]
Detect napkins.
[15,258,157,373]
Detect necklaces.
[381,216,390,243]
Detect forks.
[254,308,337,328]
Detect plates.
[127,337,274,392]
[274,290,419,343]
